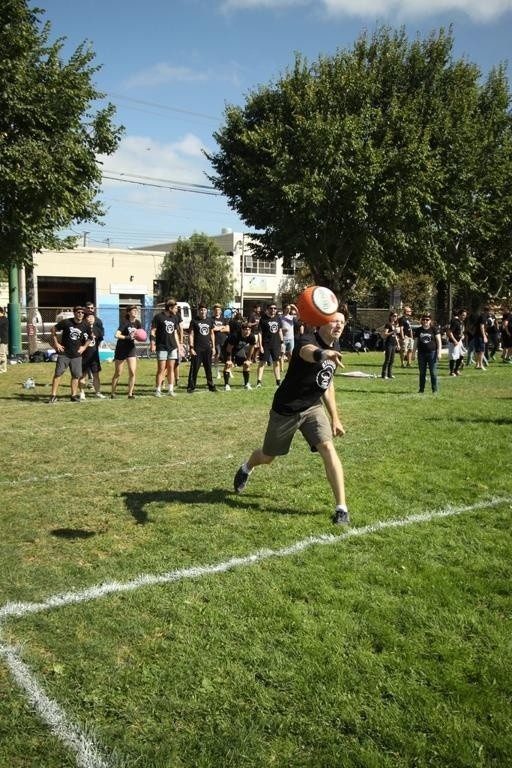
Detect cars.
[340,320,383,349]
[409,323,422,337]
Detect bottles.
[25,377,35,389]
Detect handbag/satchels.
[6,349,58,366]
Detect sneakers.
[95,391,107,400]
[207,381,218,392]
[224,383,232,392]
[127,395,136,400]
[233,463,255,492]
[243,383,253,389]
[46,395,58,404]
[381,346,512,395]
[333,508,350,526]
[70,394,81,403]
[186,386,195,394]
[167,390,176,397]
[79,391,87,400]
[256,382,262,388]
[110,394,117,400]
[155,391,163,398]
[216,370,234,379]
[79,379,94,390]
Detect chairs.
[134,342,151,360]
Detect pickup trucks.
[20,310,64,350]
[151,301,262,363]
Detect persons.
[212,304,318,390]
[232,303,354,525]
[356,325,385,353]
[381,306,512,395]
[49,298,218,403]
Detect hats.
[73,305,85,312]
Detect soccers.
[135,329,147,341]
[297,286,339,327]
